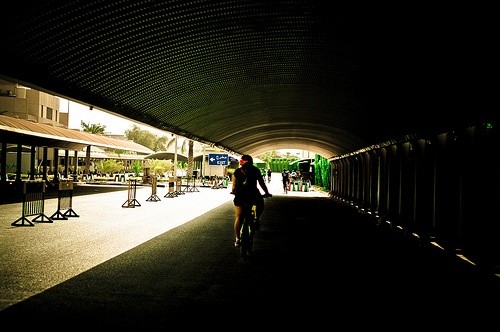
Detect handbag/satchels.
[234,178,248,206]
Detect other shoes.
[254,219,260,231]
[234,240,241,248]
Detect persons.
[281,165,316,191]
[258,165,274,183]
[232,154,270,250]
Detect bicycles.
[230,192,273,267]
